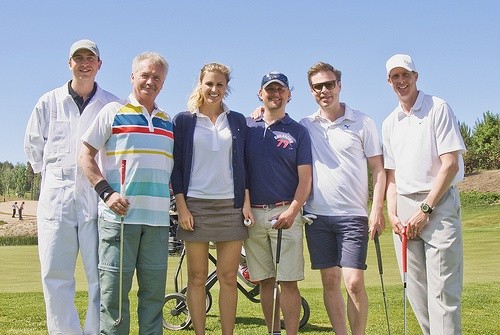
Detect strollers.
[162,195,310,330]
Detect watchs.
[420,202,433,214]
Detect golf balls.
[244,218,252,227]
[270,219,278,228]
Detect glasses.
[311,78,340,90]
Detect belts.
[251,203,291,210]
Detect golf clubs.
[402,226,408,335]
[374,231,391,335]
[112,160,126,327]
[271,229,283,335]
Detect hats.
[386,54,416,79]
[261,72,289,88]
[70,40,100,57]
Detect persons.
[18,202,24,220]
[76,51,174,335]
[24,39,120,335]
[298,62,387,335]
[11,202,19,218]
[172,63,255,335]
[243,70,312,335]
[381,53,466,335]
